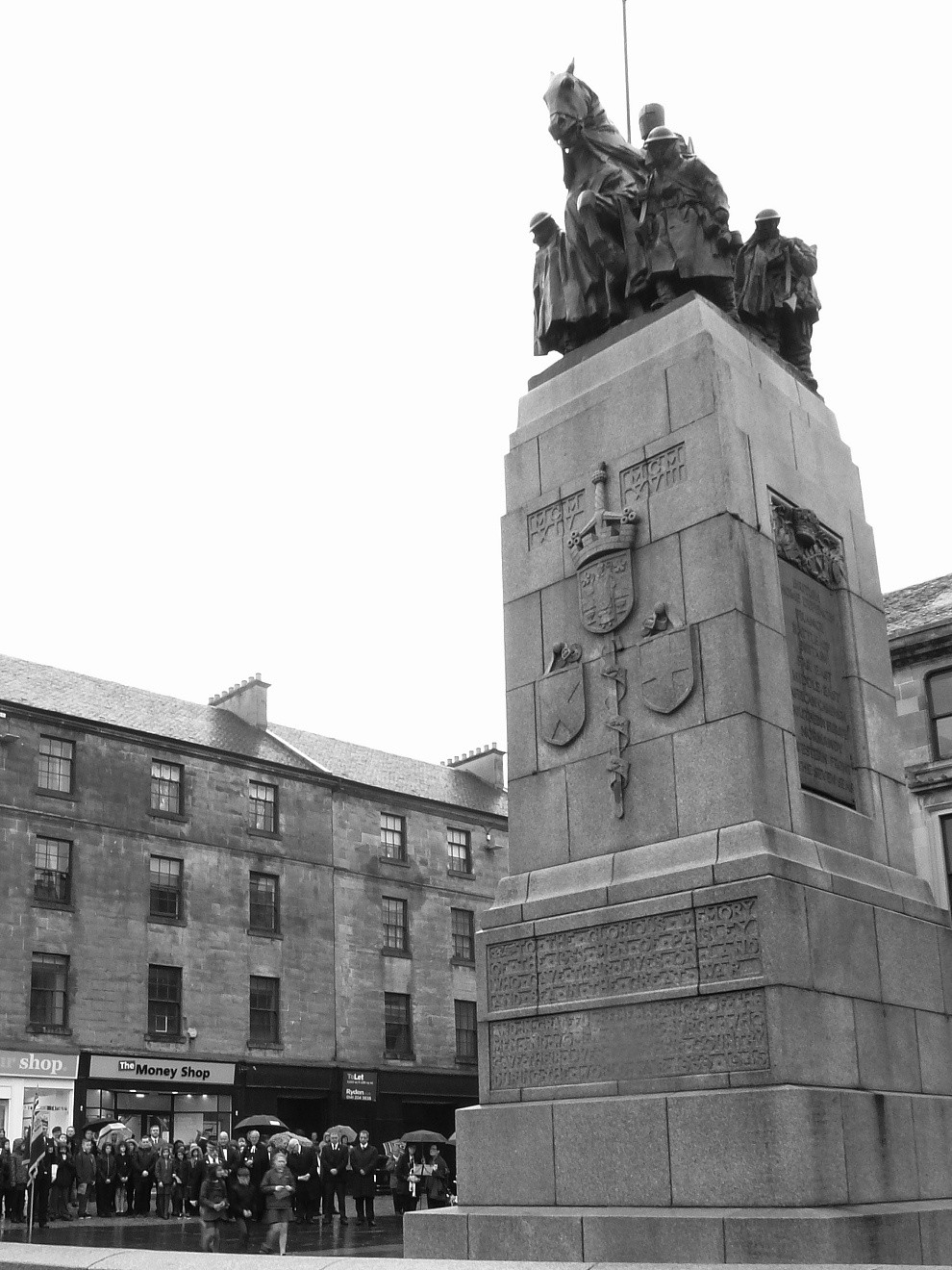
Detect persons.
[227,1167,261,1254]
[421,1144,450,1210]
[260,1151,297,1256]
[32,877,59,901]
[522,104,823,393]
[198,1162,229,1252]
[384,1145,409,1217]
[394,1143,424,1215]
[0,1126,381,1229]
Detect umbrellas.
[446,1132,457,1146]
[398,1130,449,1159]
[233,1115,289,1136]
[79,1116,118,1133]
[324,1124,358,1143]
[383,1138,409,1160]
[96,1122,135,1150]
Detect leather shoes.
[0,1196,456,1257]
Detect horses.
[542,57,739,322]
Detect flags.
[26,1089,48,1188]
